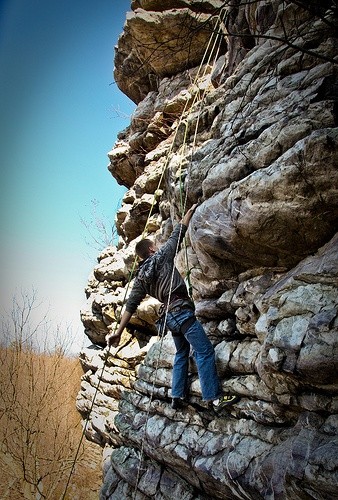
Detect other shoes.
[213,393,239,413]
[171,394,195,410]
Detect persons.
[107,203,240,414]
